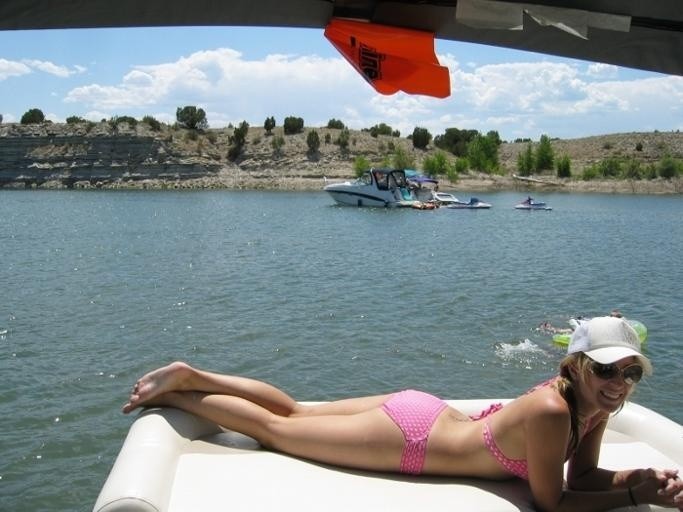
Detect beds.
[86,385,680,512]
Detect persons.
[122,316,683,512]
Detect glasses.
[589,361,645,385]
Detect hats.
[567,315,653,377]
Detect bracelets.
[627,486,638,508]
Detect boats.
[320,165,440,209]
[552,318,650,350]
[406,169,458,204]
[448,199,492,209]
[512,196,547,210]
